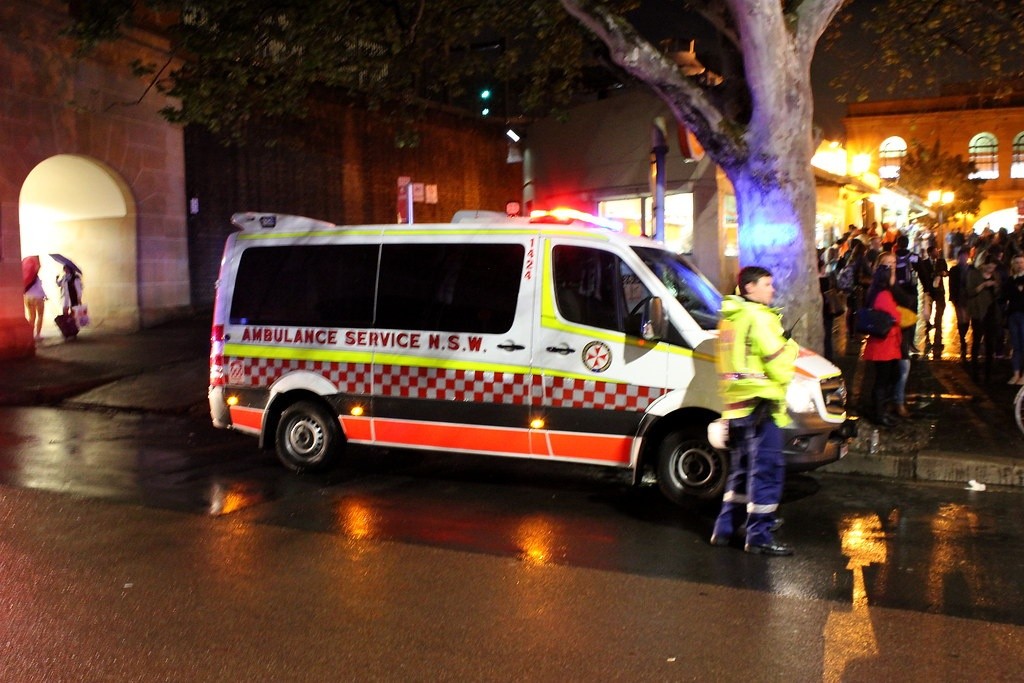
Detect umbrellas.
[46,252,82,283]
[21,252,41,295]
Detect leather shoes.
[710,527,746,547]
[744,540,794,557]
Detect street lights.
[929,187,956,261]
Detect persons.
[813,200,1024,432]
[54,266,85,332]
[24,274,49,341]
[702,265,803,556]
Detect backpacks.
[836,260,860,292]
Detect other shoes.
[873,414,898,427]
[897,403,913,417]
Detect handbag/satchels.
[54,314,80,339]
[72,302,91,329]
[853,307,896,340]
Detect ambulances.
[208,211,849,512]
